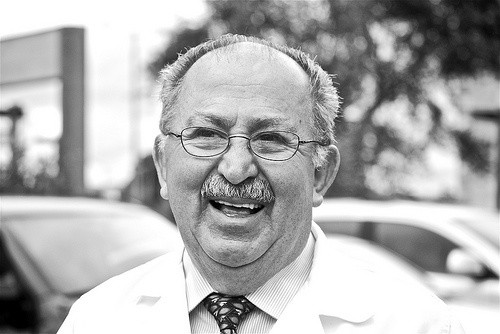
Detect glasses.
[165,126,322,162]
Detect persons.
[54,35,452,334]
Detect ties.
[201,291,257,334]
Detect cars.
[307,199,500,314]
[1,195,185,334]
